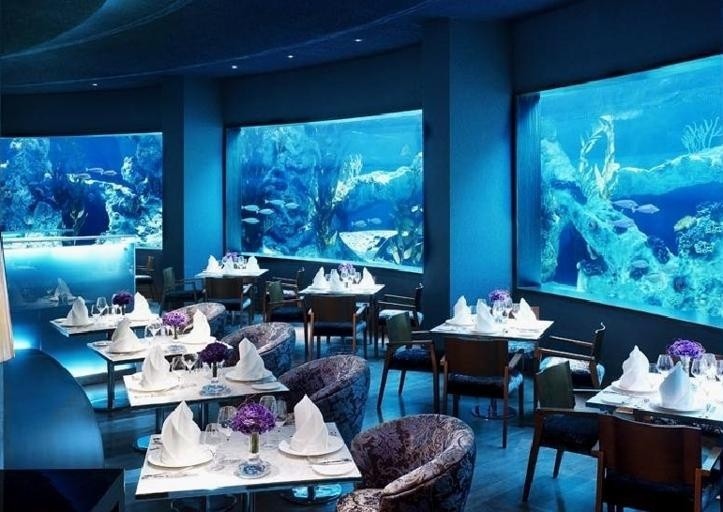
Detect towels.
[289,394,328,453]
[660,361,692,408]
[621,345,651,388]
[158,400,206,465]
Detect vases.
[249,433,261,455]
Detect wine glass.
[89,294,124,326]
[324,267,361,289]
[216,255,247,272]
[201,396,289,473]
[476,297,521,323]
[142,316,171,346]
[169,351,211,389]
[657,350,723,389]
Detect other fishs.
[610,200,638,210]
[403,246,412,259]
[411,204,421,213]
[631,204,660,214]
[351,220,368,228]
[10,141,22,148]
[607,219,635,229]
[391,246,400,264]
[367,218,383,225]
[240,199,299,223]
[631,260,649,268]
[73,167,116,180]
[44,173,52,180]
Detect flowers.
[229,404,278,453]
[667,338,705,364]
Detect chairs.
[273,355,370,447]
[438,337,524,450]
[519,361,609,509]
[594,413,718,512]
[343,410,476,510]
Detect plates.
[276,429,356,477]
[148,446,210,467]
[610,373,706,414]
[224,361,281,390]
[54,316,96,328]
[181,334,217,345]
[445,314,546,336]
[127,367,178,396]
[92,340,146,354]
